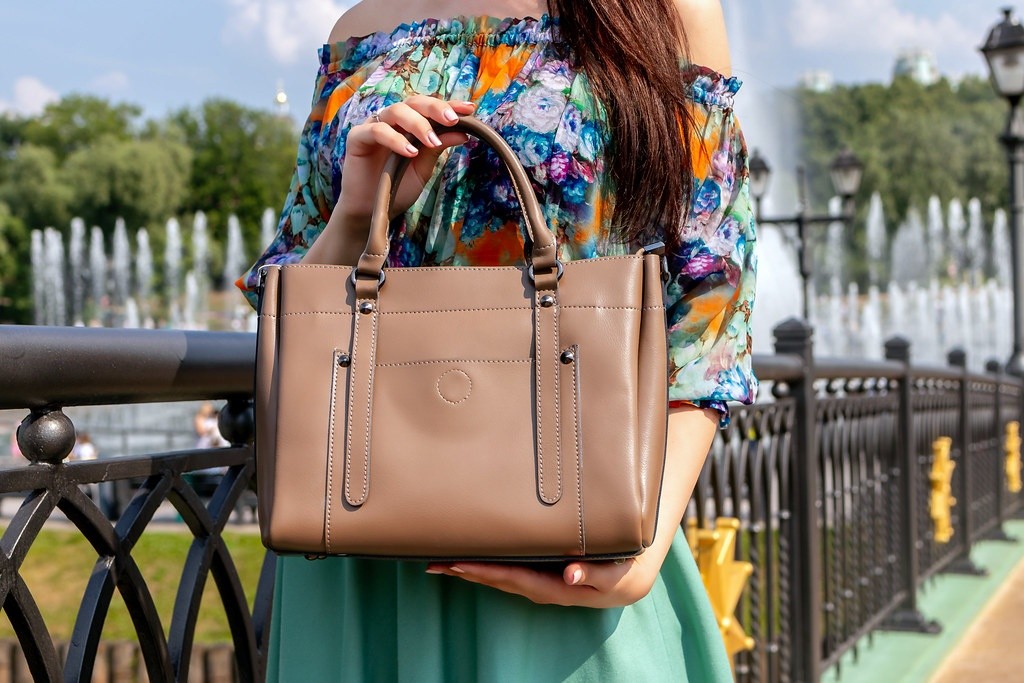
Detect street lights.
[978,7,1024,379]
[749,147,861,319]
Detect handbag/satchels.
[253,115,670,565]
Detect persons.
[232,0,756,682]
